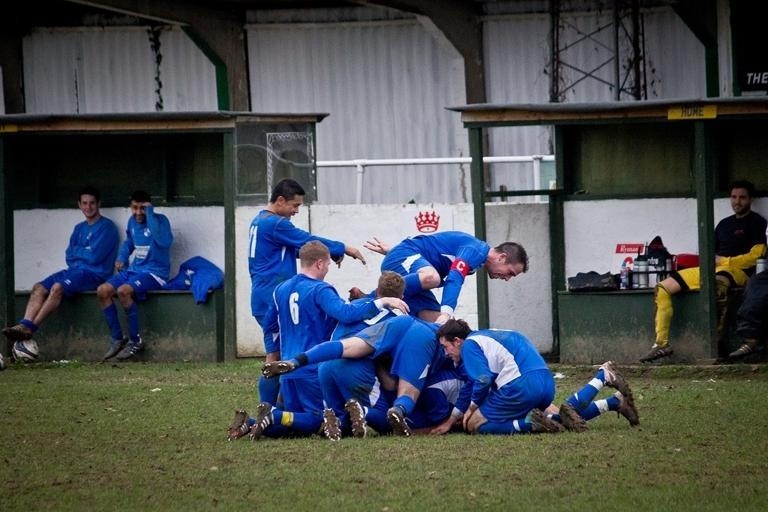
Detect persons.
[1,186,121,341]
[638,179,768,363]
[226,231,639,440]
[725,270,768,358]
[248,178,366,408]
[95,190,174,360]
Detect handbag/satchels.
[568,272,616,291]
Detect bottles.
[631,256,648,289]
[755,254,766,275]
[618,261,629,290]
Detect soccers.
[12,340,39,363]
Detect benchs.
[13,289,224,364]
[556,288,746,364]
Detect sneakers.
[639,345,673,363]
[262,360,296,379]
[116,335,143,360]
[228,398,413,442]
[729,339,760,358]
[1,325,32,339]
[104,336,128,360]
[524,360,640,432]
